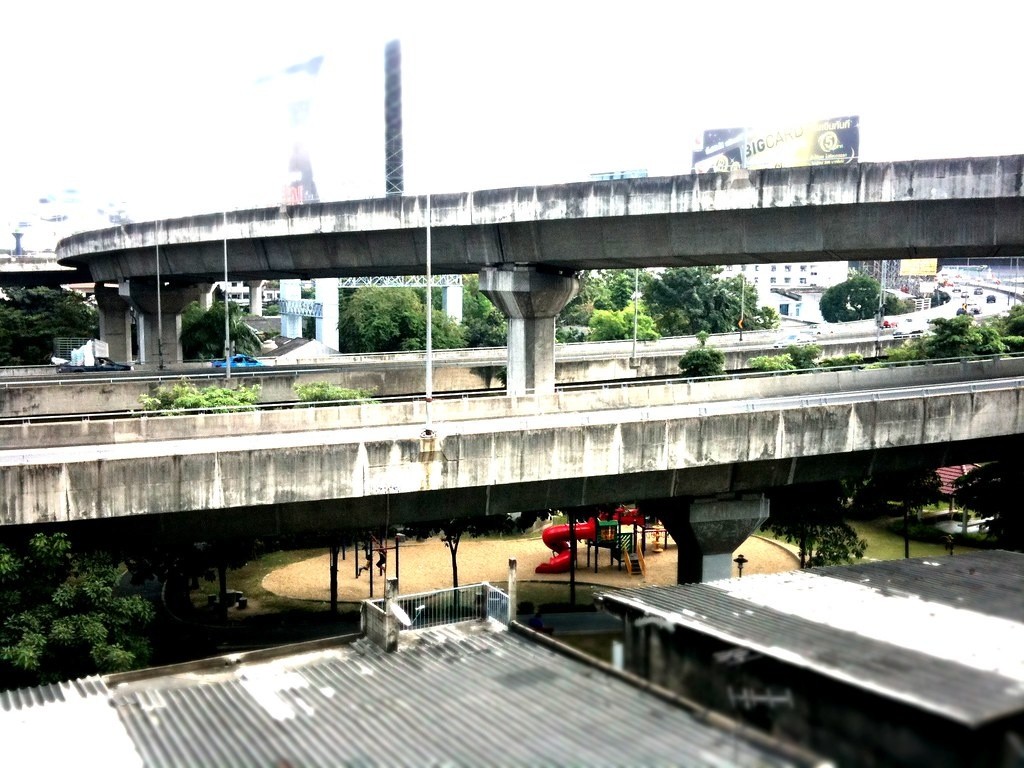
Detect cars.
[986,294,997,303]
[974,287,985,295]
[212,352,266,368]
[939,276,981,316]
[883,318,898,329]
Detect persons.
[358,554,370,576]
[376,549,386,572]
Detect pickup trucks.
[56,357,132,372]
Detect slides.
[535,516,595,574]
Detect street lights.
[733,554,747,576]
